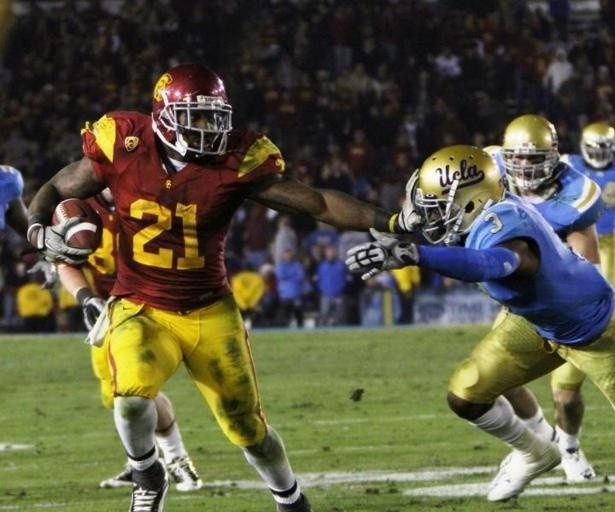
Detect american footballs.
[51,198,103,254]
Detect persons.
[1,163,85,332]
[558,123,615,281]
[226,198,422,327]
[0,0,232,206]
[51,187,205,493]
[232,0,615,208]
[344,145,615,504]
[26,64,428,512]
[475,113,606,481]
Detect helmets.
[413,146,503,246]
[485,143,502,159]
[150,70,233,166]
[498,115,560,190]
[579,123,615,169]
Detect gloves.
[397,170,423,231]
[81,295,104,331]
[344,227,419,280]
[27,261,57,288]
[26,219,90,268]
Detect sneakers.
[488,443,563,502]
[100,465,138,490]
[127,477,171,512]
[167,454,202,491]
[560,439,597,484]
[548,422,561,442]
